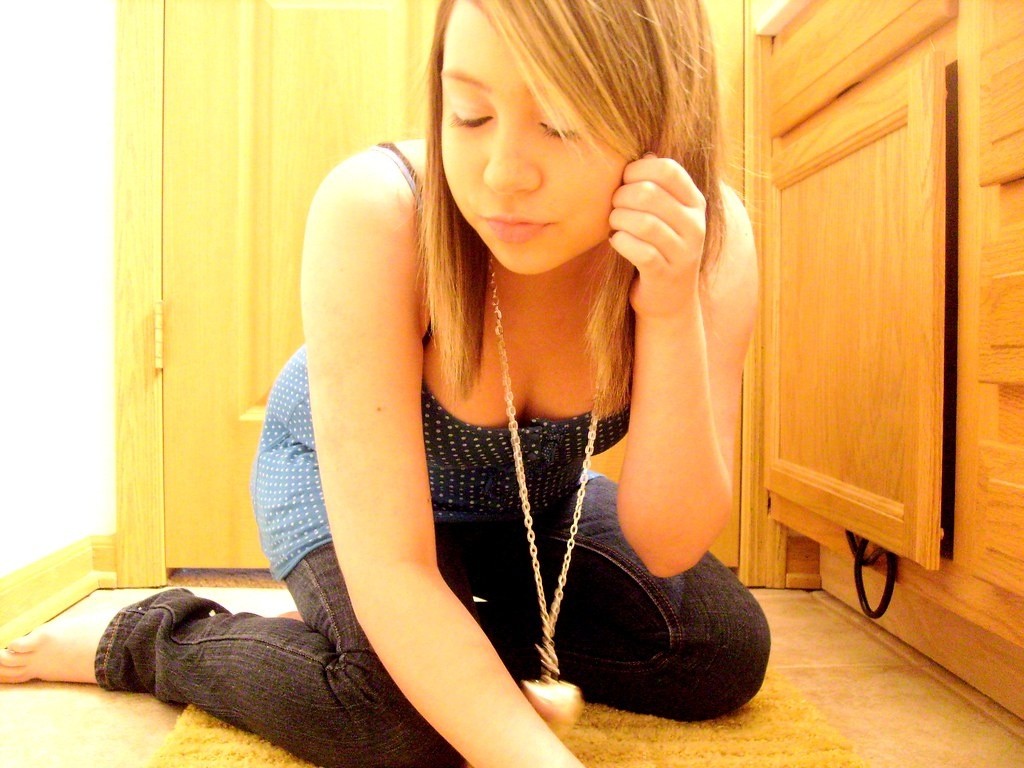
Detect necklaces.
[484,248,610,744]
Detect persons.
[0,0,770,768]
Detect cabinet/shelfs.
[764,1,961,587]
